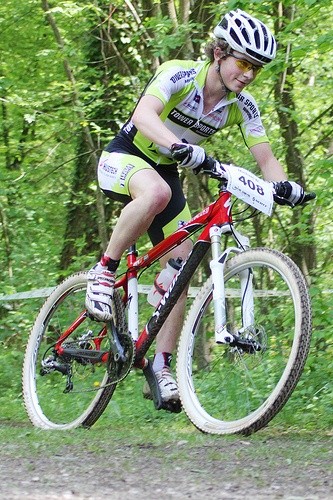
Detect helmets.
[214,9,277,64]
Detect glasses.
[227,52,265,74]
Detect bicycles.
[20,142,316,439]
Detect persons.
[85,8,306,406]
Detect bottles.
[147,257,183,308]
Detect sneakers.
[86,262,120,322]
[142,369,180,404]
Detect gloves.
[281,180,305,208]
[169,143,205,170]
